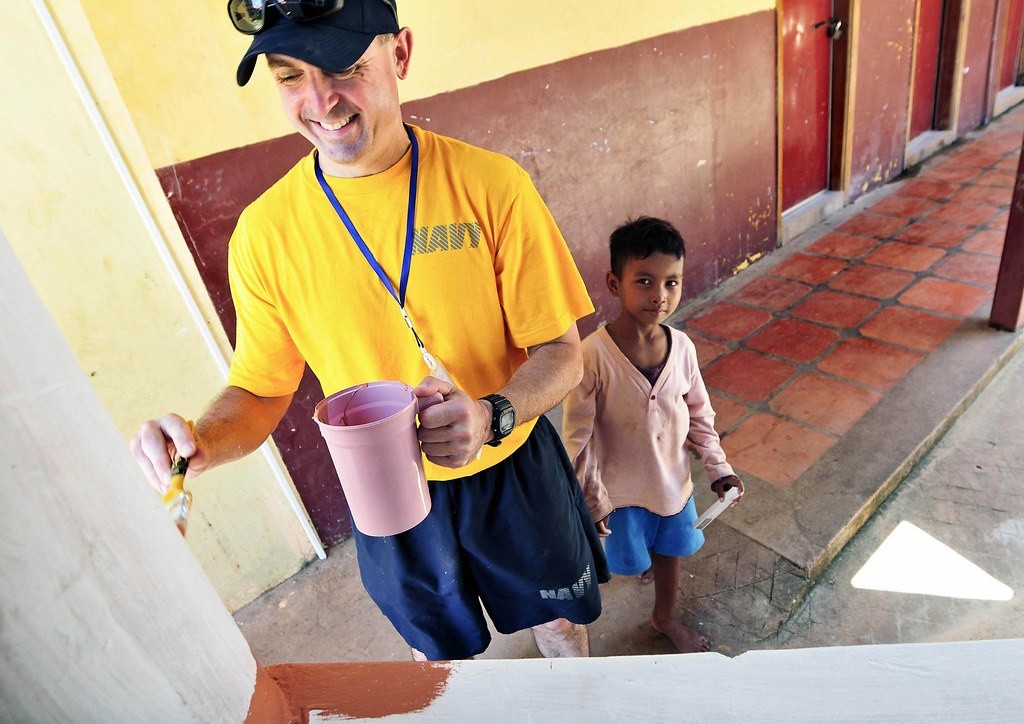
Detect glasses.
[227,0,345,35]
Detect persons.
[560,207,745,655]
[130,1,613,659]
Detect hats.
[236,0,400,87]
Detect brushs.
[160,420,195,536]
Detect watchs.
[477,393,516,448]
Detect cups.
[313,380,442,538]
